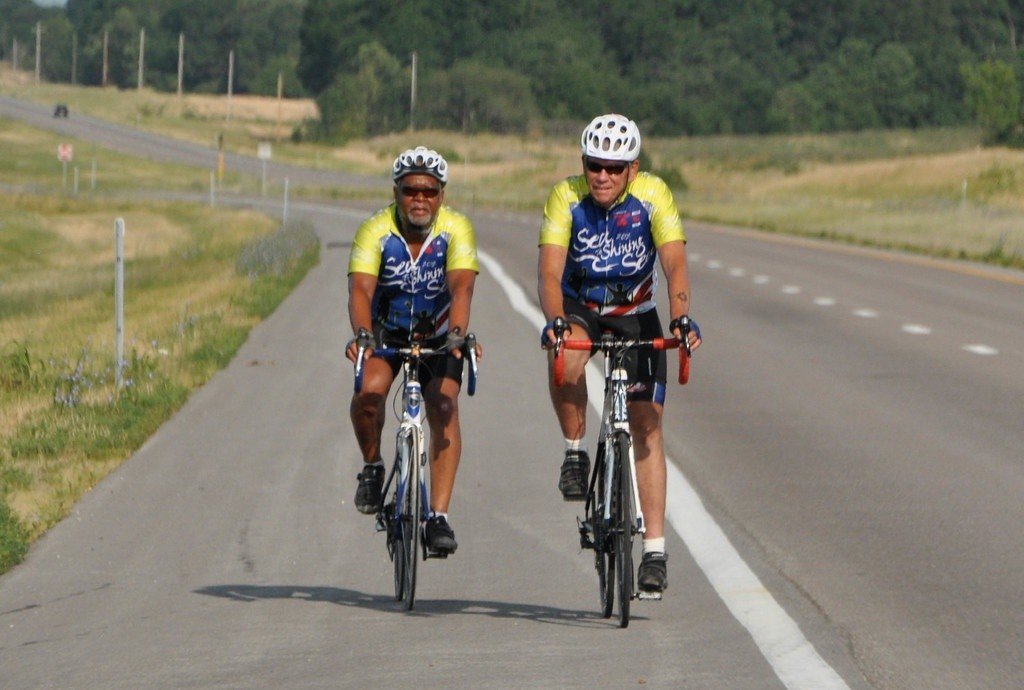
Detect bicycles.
[549,316,692,628]
[351,327,478,608]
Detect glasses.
[583,160,627,174]
[403,186,440,197]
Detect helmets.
[580,112,641,162]
[392,145,449,183]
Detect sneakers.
[558,449,591,496]
[637,550,668,593]
[425,515,458,554]
[353,464,386,515]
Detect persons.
[537,114,702,591]
[346,145,480,552]
